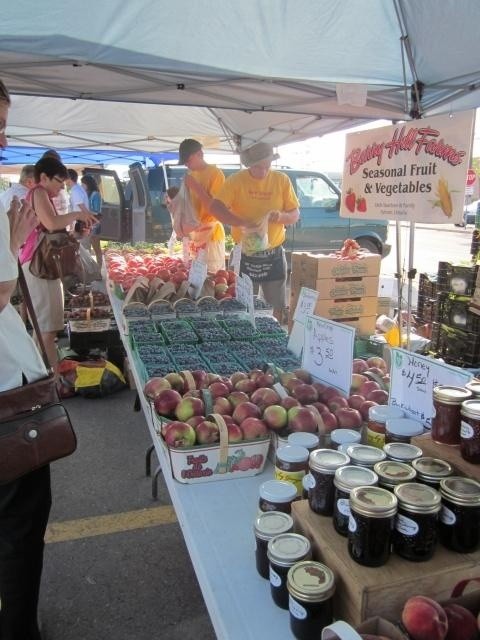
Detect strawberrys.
[345,187,357,213]
[355,198,367,213]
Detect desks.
[100,240,480,640]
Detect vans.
[80,162,392,280]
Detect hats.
[240,143,280,167]
[177,139,203,166]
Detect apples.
[106,252,239,299]
[145,356,390,448]
[238,458,251,470]
[251,454,263,468]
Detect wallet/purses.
[75,214,103,232]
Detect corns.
[428,178,461,219]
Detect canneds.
[253,381,480,640]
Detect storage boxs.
[67,317,120,353]
[287,248,383,354]
[123,358,136,392]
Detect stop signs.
[465,170,477,185]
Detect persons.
[1,82,78,639]
[209,142,300,326]
[162,138,226,277]
[0,148,103,397]
[164,187,189,268]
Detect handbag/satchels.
[0,373,77,485]
[55,356,128,398]
[29,229,79,280]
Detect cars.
[454,202,480,227]
